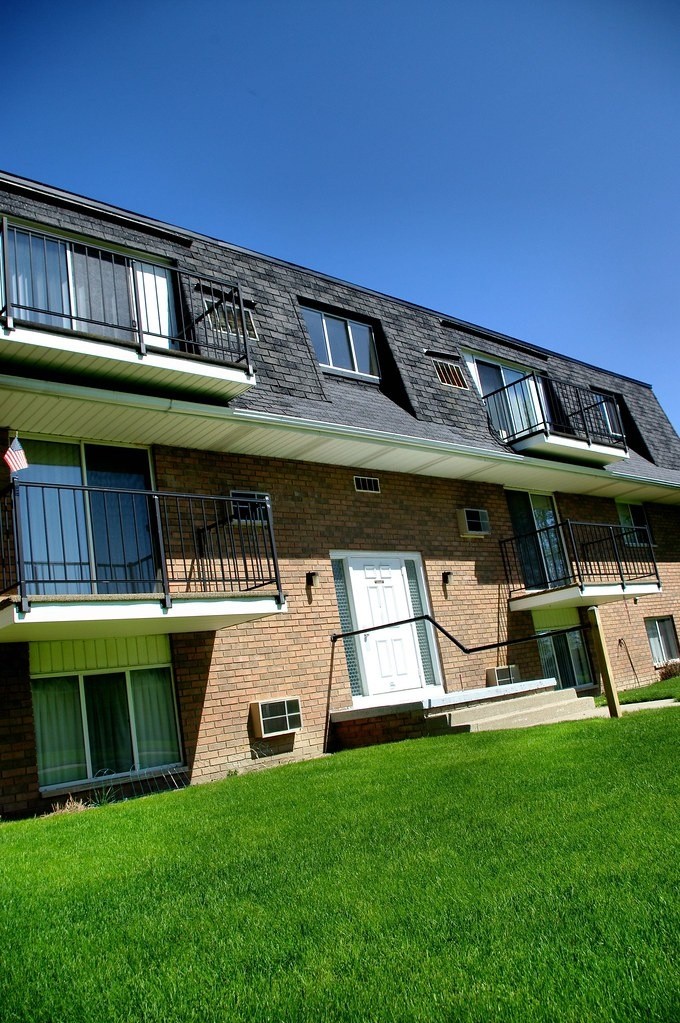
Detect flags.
[2,438,29,474]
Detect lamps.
[306,571,319,587]
[440,572,453,584]
